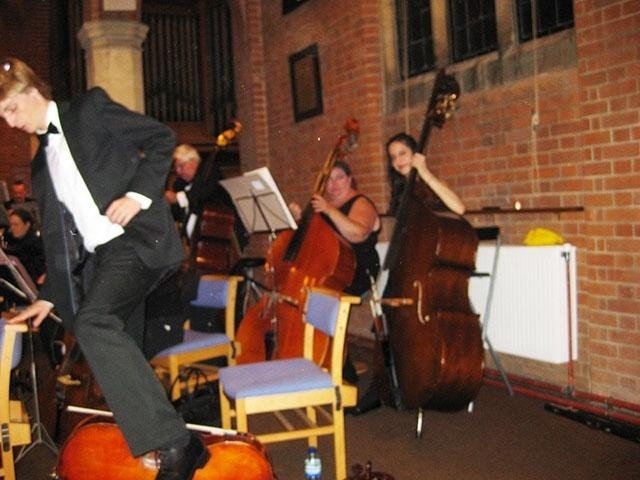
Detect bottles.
[304,445,324,480]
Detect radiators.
[375,241,579,365]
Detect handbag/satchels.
[171,389,220,427]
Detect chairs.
[467,226,515,412]
[0,317,32,480]
[218,286,362,480]
[150,275,244,400]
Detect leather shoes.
[153,433,210,479]
[352,397,380,415]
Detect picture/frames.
[288,43,324,123]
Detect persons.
[1,205,49,310]
[343,132,466,416]
[308,158,382,386]
[1,55,212,480]
[3,179,33,210]
[163,141,250,254]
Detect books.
[219,166,299,233]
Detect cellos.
[233,119,358,374]
[384,70,484,412]
[53,415,394,480]
[146,121,243,316]
[41,325,107,443]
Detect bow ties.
[37,122,58,147]
